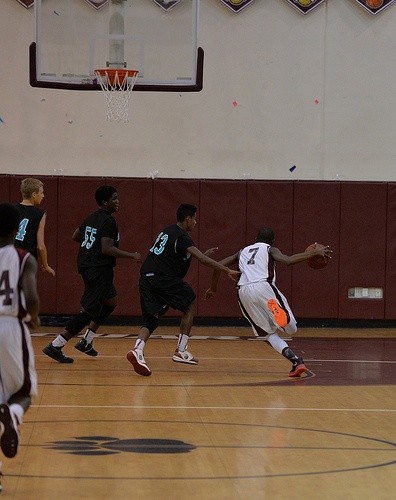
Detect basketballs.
[304,244,328,268]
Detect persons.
[127,203,242,376]
[14,178,55,279]
[42,185,141,363]
[0,202,39,491]
[204,228,333,377]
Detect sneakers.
[0,403,19,458]
[75,338,98,357]
[42,343,73,363]
[289,358,306,377]
[267,299,288,327]
[172,348,198,365]
[127,350,151,376]
